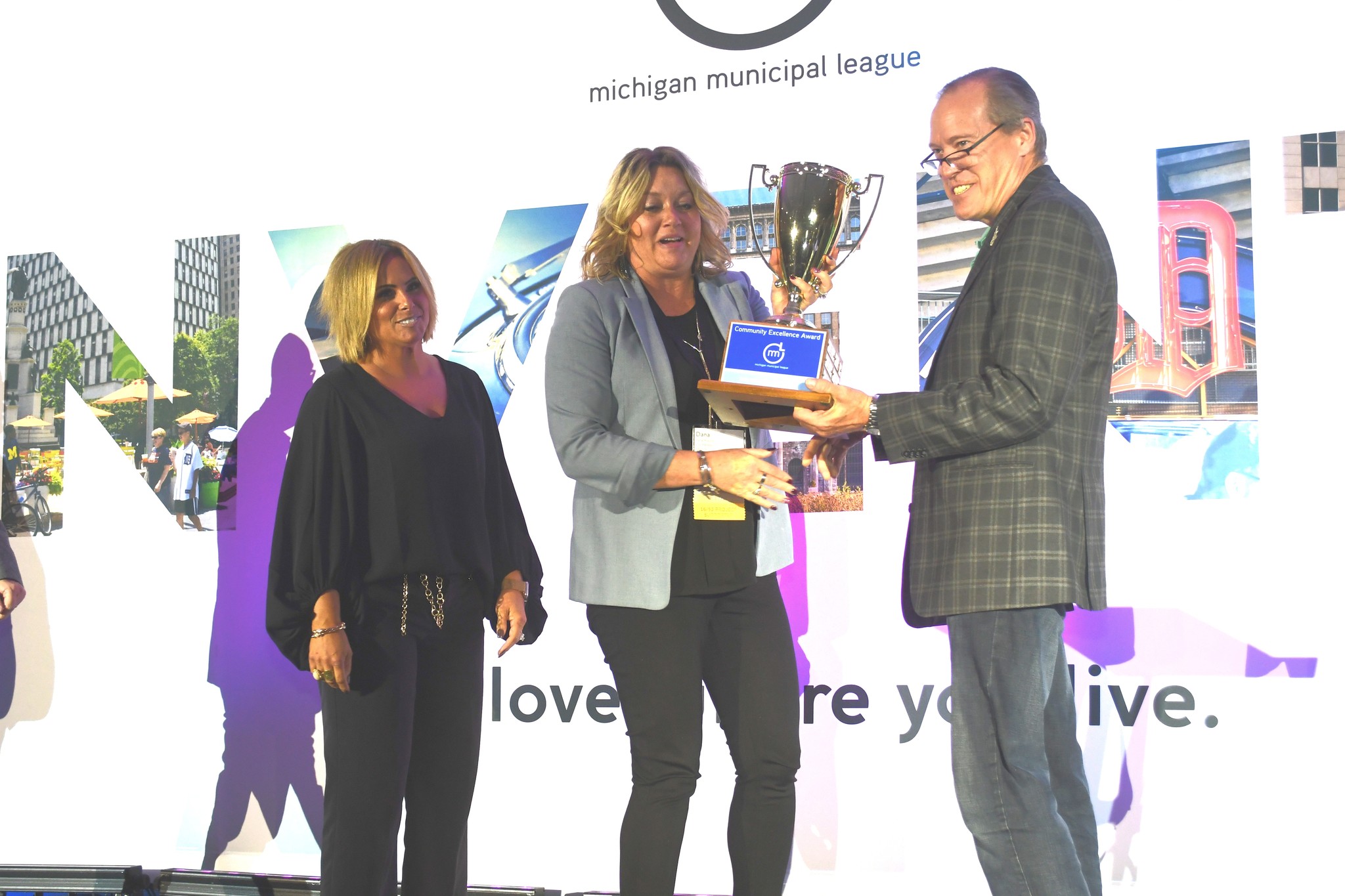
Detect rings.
[817,290,828,299]
[323,670,334,681]
[753,486,761,495]
[759,473,767,485]
[312,669,323,680]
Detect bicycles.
[2,472,39,537]
[2,467,52,536]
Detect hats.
[176,422,192,430]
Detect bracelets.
[310,622,347,639]
[698,449,719,496]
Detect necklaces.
[677,307,719,431]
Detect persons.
[4,379,231,537]
[546,145,840,896]
[265,239,547,896]
[0,518,25,620]
[789,67,1117,895]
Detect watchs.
[500,577,529,605]
[862,393,882,436]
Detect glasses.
[152,436,162,439]
[177,430,186,435]
[920,121,1007,177]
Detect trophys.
[706,160,885,419]
